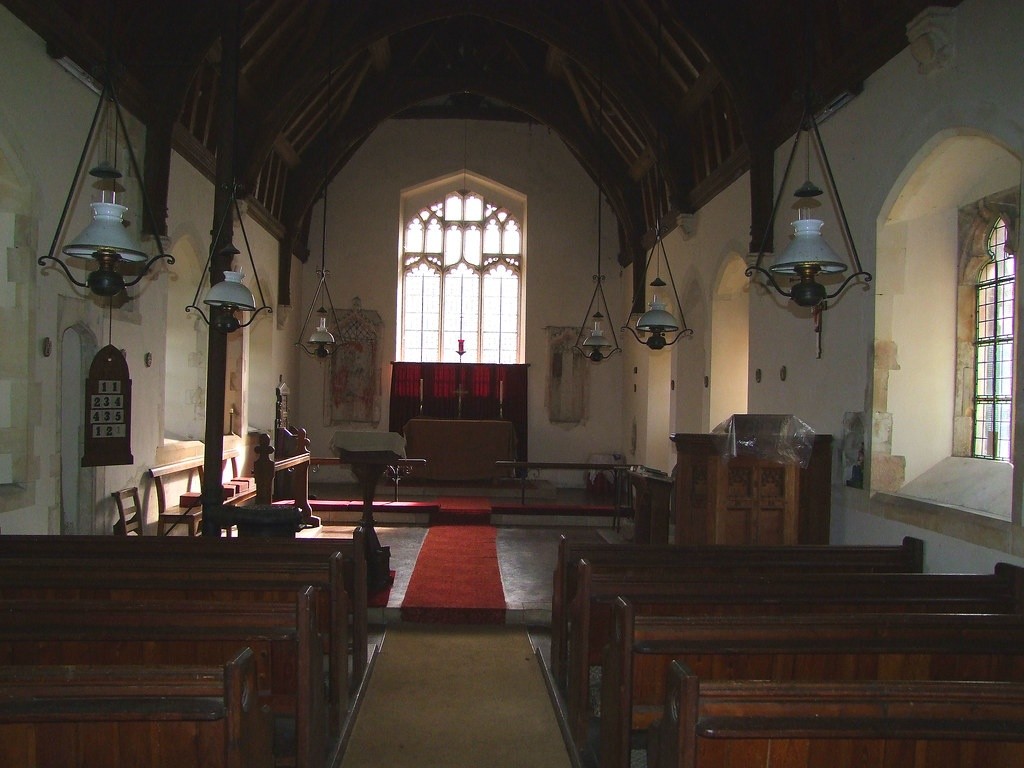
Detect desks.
[402,416,519,477]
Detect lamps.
[619,162,694,350]
[293,0,349,361]
[573,80,622,365]
[184,6,273,334]
[743,3,874,309]
[39,0,175,297]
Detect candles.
[420,378,424,403]
[500,381,503,404]
[458,339,465,352]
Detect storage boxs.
[180,476,256,509]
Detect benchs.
[311,458,426,502]
[0,534,370,768]
[497,462,633,508]
[149,450,258,537]
[552,535,1023,768]
